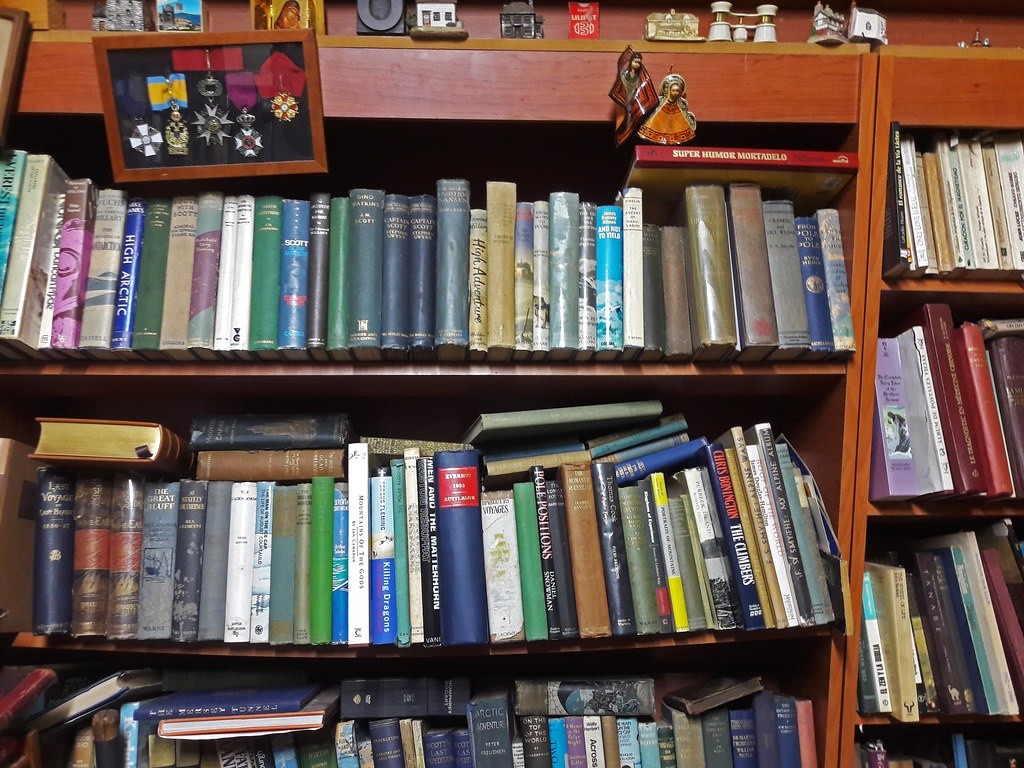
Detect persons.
[610,46,695,144]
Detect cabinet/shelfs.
[0,30,880,768]
[839,46,1024,768]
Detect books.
[0,661,822,768]
[0,145,859,364]
[851,731,1024,768]
[871,296,1024,506]
[880,118,1024,284]
[857,516,1024,723]
[0,393,848,653]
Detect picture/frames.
[0,6,29,146]
[92,28,328,183]
